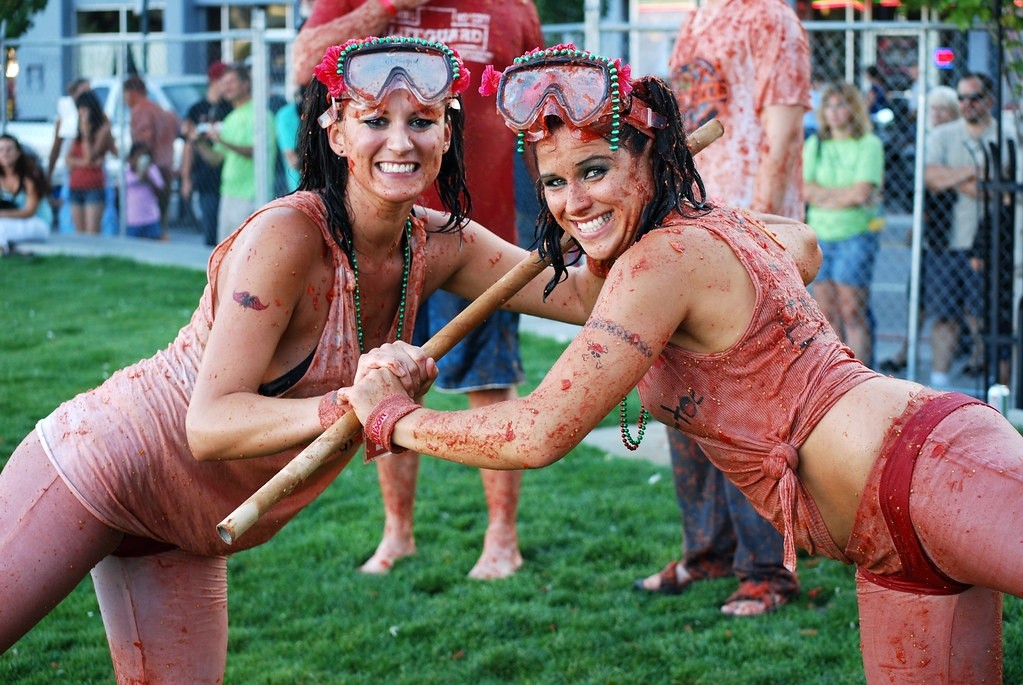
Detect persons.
[292,0,546,576]
[337,50,1023,684]
[0,38,824,685]
[631,0,812,616]
[0,63,1023,396]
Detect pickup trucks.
[6,76,215,198]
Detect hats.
[210,62,229,80]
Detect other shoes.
[880,357,907,372]
[961,363,984,377]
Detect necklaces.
[343,205,410,360]
[614,377,651,453]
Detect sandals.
[721,582,792,617]
[633,559,698,591]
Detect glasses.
[337,37,453,108]
[956,89,989,103]
[497,56,613,132]
